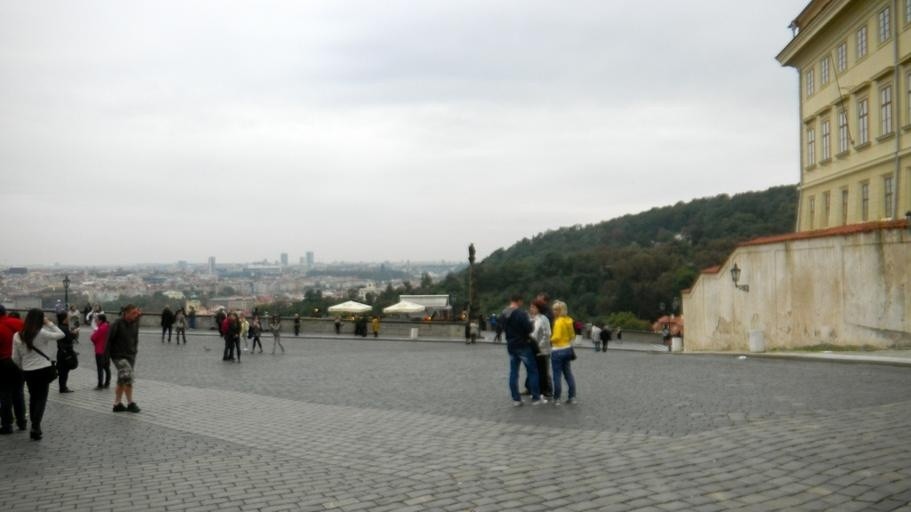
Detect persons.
[1,302,142,442]
[175,308,190,345]
[161,304,175,343]
[465,291,622,407]
[181,307,188,318]
[335,315,343,335]
[352,314,379,337]
[662,325,669,343]
[217,304,287,363]
[293,312,300,335]
[189,306,197,328]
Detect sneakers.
[513,398,525,407]
[566,397,578,405]
[126,402,140,413]
[518,386,531,395]
[529,392,560,407]
[113,402,127,412]
[16,423,26,431]
[0,425,13,435]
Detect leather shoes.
[30,431,41,441]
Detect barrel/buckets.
[409,328,419,339]
[671,337,683,351]
[748,329,764,353]
[576,334,584,344]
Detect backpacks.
[62,351,78,370]
[245,322,254,338]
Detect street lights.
[62,275,73,307]
[659,301,679,331]
[468,243,476,319]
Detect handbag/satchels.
[570,348,575,360]
[47,360,58,384]
[176,317,185,328]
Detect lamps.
[728,259,749,294]
